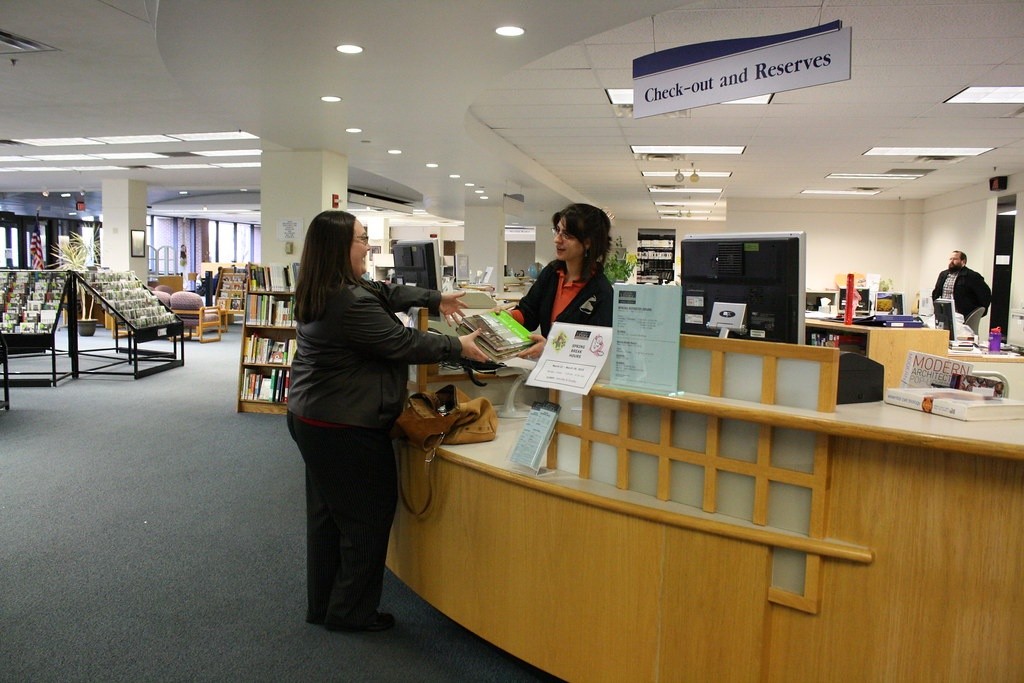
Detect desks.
[805,291,837,310]
[805,317,949,396]
[949,352,1023,403]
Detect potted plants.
[45,223,103,337]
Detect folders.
[852,314,913,323]
[857,321,923,328]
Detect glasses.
[550,227,576,240]
[352,233,369,246]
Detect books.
[0,272,67,334]
[82,271,175,328]
[217,276,243,310]
[886,387,1024,421]
[241,262,300,402]
[456,310,535,363]
[949,340,973,350]
[637,240,673,283]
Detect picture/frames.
[131,229,146,258]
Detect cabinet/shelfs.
[237,263,299,414]
[214,267,247,332]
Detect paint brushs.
[931,383,950,388]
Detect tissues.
[818,297,837,314]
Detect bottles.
[988,326,1001,352]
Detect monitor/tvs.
[393,238,443,293]
[888,292,903,315]
[839,287,869,318]
[806,291,837,312]
[680,231,806,346]
[934,298,956,340]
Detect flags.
[31,214,44,270]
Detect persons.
[495,204,614,358]
[214,267,223,278]
[286,210,489,634]
[931,251,991,322]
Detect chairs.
[148,286,221,343]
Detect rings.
[528,355,529,358]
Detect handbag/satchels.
[392,384,498,516]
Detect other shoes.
[306,613,398,632]
